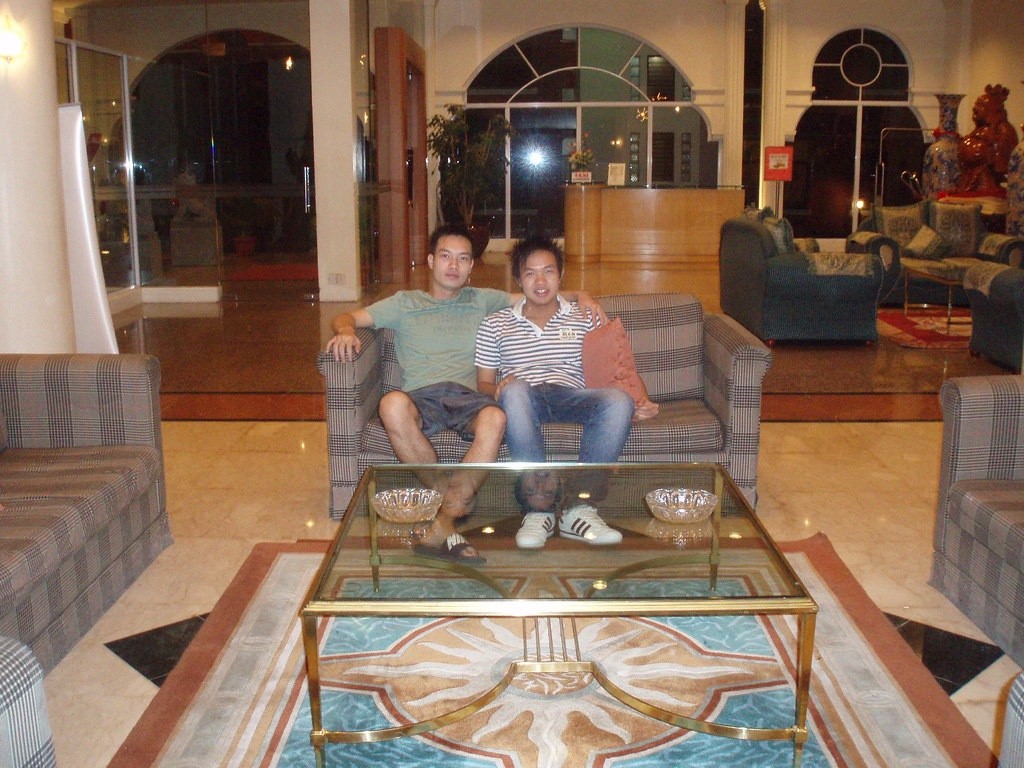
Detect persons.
[514,469,564,513]
[326,225,612,559]
[475,234,660,548]
[433,465,478,527]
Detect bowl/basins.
[645,488,718,524]
[645,516,715,548]
[371,487,443,522]
[375,517,441,544]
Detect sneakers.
[559,506,623,544]
[515,510,557,549]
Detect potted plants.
[409,105,513,261]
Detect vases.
[571,165,594,184]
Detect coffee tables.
[299,461,818,768]
[903,266,974,326]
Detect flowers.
[569,135,597,167]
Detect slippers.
[413,532,487,566]
[453,515,469,527]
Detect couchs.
[714,207,883,342]
[844,200,1023,370]
[928,376,1024,768]
[318,292,774,518]
[1,350,174,768]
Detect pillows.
[874,202,927,257]
[585,314,660,423]
[764,217,797,254]
[747,207,774,222]
[928,203,982,258]
[904,226,948,262]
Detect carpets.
[107,529,994,768]
[874,304,974,349]
